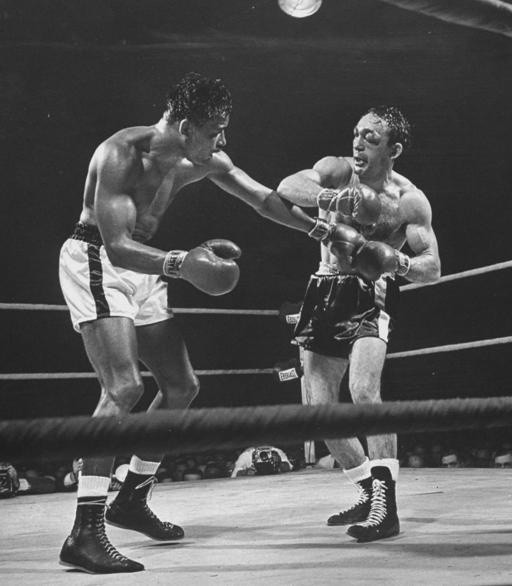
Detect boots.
[327,466,400,540]
[58,494,144,574]
[104,469,185,541]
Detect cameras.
[0,465,13,497]
[251,444,278,475]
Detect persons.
[57,68,376,573]
[0,456,130,499]
[274,104,441,541]
[403,443,512,469]
[230,446,294,478]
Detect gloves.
[162,239,241,298]
[308,182,410,280]
